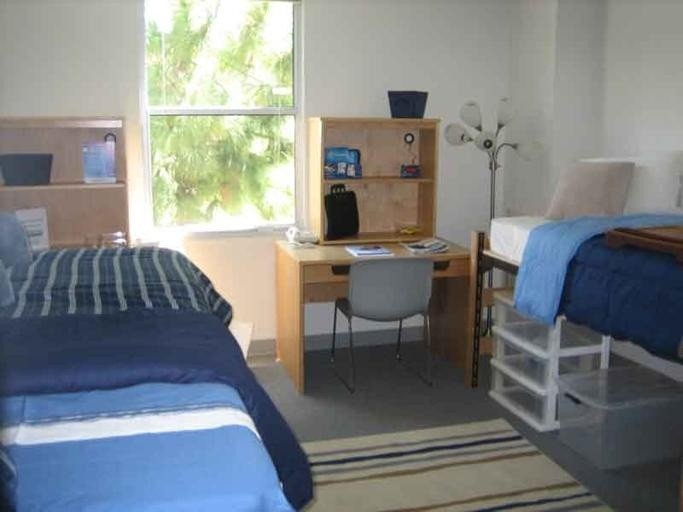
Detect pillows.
[543,160,636,221]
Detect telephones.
[285,226,319,244]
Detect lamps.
[443,97,539,337]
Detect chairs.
[329,256,433,394]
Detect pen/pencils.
[359,246,381,250]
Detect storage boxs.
[553,364,683,471]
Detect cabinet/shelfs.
[306,116,441,246]
[0,118,130,246]
[487,289,610,434]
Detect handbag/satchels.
[325,184,359,240]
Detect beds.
[481,212,683,440]
[0,212,312,512]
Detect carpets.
[293,418,615,512]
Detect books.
[345,244,395,258]
[15,207,50,251]
[399,238,450,253]
[82,142,117,183]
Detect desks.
[276,237,475,396]
